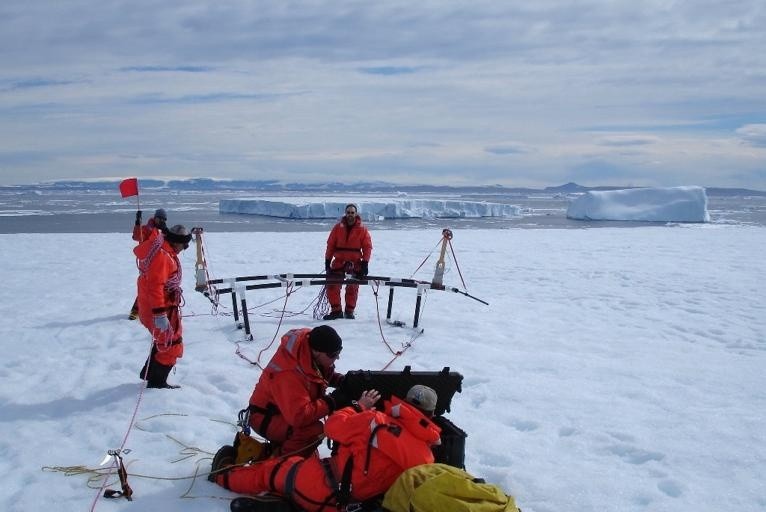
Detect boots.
[232,432,266,466]
[142,346,181,389]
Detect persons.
[233,325,344,464]
[322,203,371,321]
[208,383,442,512]
[127,206,168,321]
[135,223,192,391]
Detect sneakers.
[209,445,236,482]
[230,493,287,512]
[129,310,138,320]
[323,311,343,320]
[344,312,354,319]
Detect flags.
[118,176,138,199]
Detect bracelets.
[352,399,362,413]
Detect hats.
[166,225,192,243]
[406,385,438,411]
[308,325,342,352]
[155,208,167,220]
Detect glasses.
[346,210,355,215]
[326,352,338,358]
[183,242,189,250]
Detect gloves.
[325,259,331,272]
[361,260,368,276]
[135,210,143,226]
[153,315,170,333]
